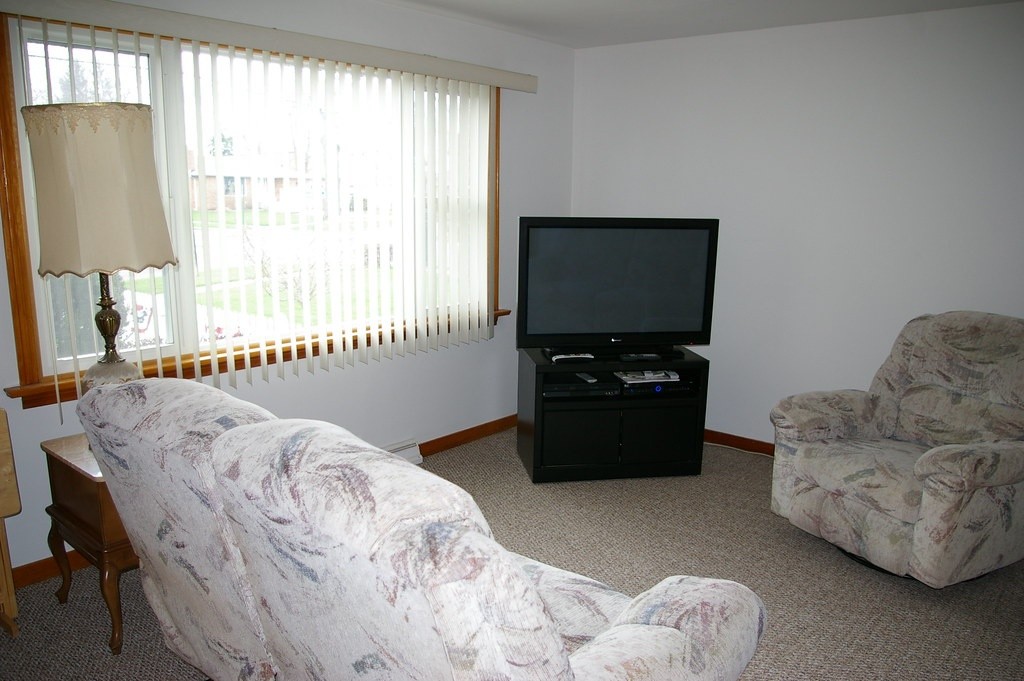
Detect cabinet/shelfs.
[517,344,711,484]
[41,429,139,656]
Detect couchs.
[770,310,1024,591]
[74,378,769,681]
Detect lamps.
[19,102,178,451]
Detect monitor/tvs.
[516,217,720,363]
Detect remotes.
[551,353,595,364]
[576,373,597,383]
